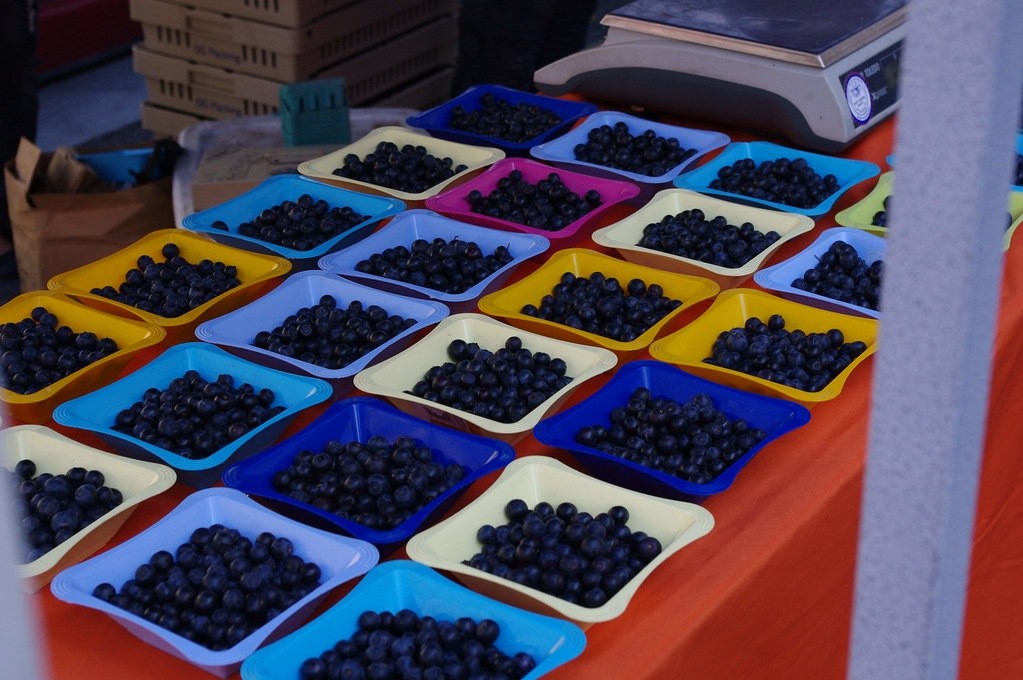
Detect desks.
[13,92,1023,680]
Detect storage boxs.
[3,0,460,292]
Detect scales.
[529,0,907,157]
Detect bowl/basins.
[0,81,1023,680]
